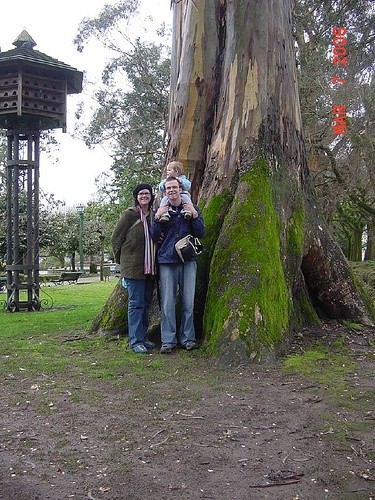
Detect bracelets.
[193,211,198,217]
[156,213,160,219]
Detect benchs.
[50,271,85,286]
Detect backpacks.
[175,234,203,263]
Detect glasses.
[137,193,149,195]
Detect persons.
[150,176,206,354]
[159,161,194,223]
[110,184,159,354]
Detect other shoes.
[184,213,191,220]
[159,213,170,222]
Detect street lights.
[75,204,86,278]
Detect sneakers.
[160,344,176,353]
[144,341,156,348]
[133,344,148,353]
[182,341,199,350]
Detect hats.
[133,184,152,198]
